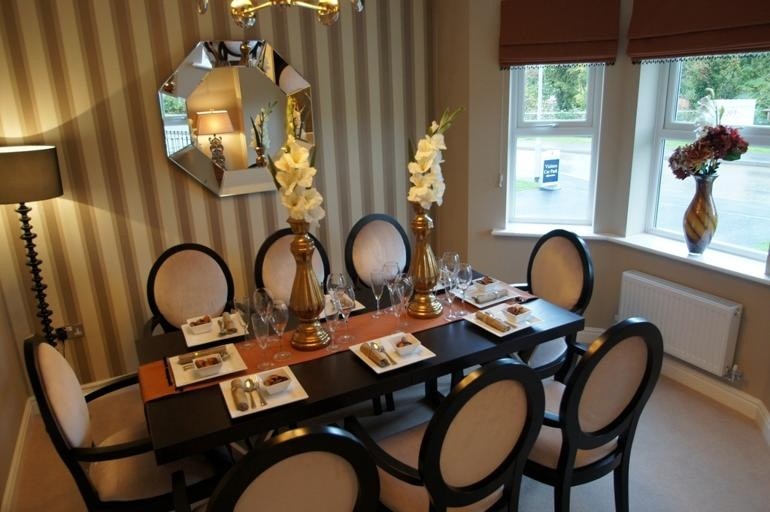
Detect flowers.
[246,102,278,146]
[267,100,327,229]
[669,124,750,182]
[405,107,465,206]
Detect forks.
[379,344,397,365]
[251,380,268,406]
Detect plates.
[449,285,520,308]
[403,274,452,292]
[349,332,436,373]
[168,344,248,388]
[463,303,544,338]
[218,366,308,419]
[181,314,249,348]
[318,293,365,318]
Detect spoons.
[242,378,257,409]
[485,312,516,329]
[216,320,226,336]
[370,342,381,353]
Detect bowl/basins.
[387,333,421,355]
[257,368,293,395]
[187,314,213,333]
[502,304,533,326]
[191,353,223,376]
[473,276,501,293]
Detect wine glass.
[233,250,473,372]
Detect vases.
[679,171,718,254]
[405,197,444,319]
[254,144,267,168]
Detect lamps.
[0,142,66,351]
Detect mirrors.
[158,39,316,197]
[192,107,234,171]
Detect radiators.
[614,267,744,381]
